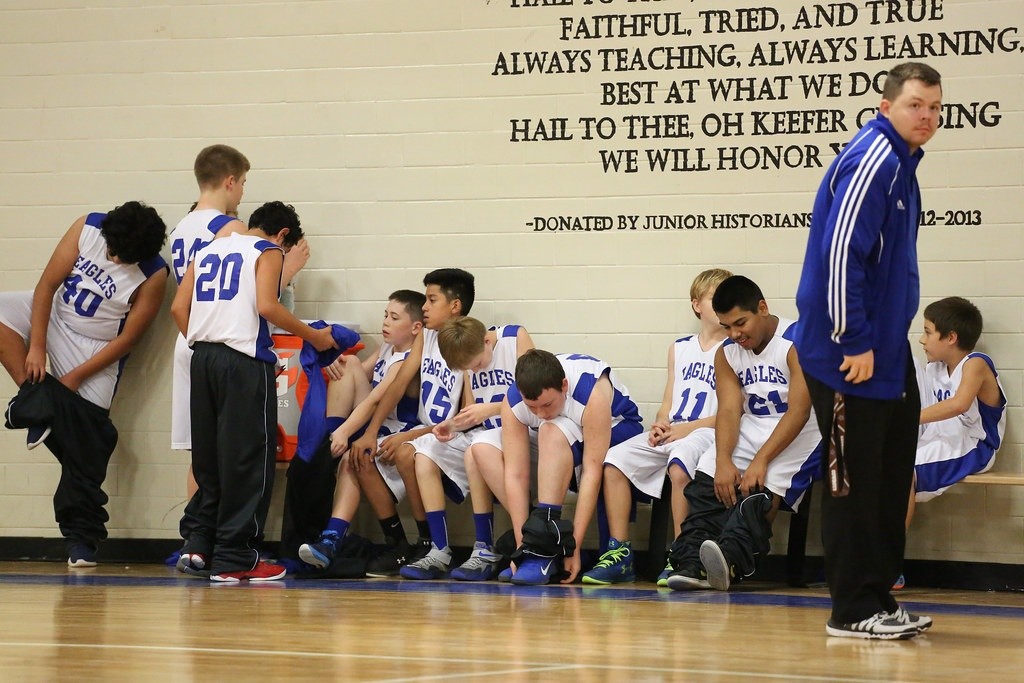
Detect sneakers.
[581,536,638,586]
[365,533,432,576]
[26,424,52,450]
[210,560,287,582]
[182,553,211,578]
[668,565,714,591]
[450,541,504,580]
[401,541,460,579]
[657,557,673,586]
[498,555,561,585]
[67,545,98,567]
[825,602,932,641]
[299,529,342,569]
[702,538,740,591]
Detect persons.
[0,200,170,567]
[298,267,643,587]
[171,143,339,585]
[795,62,944,641]
[581,268,824,591]
[888,295,1008,590]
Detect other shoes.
[893,575,906,589]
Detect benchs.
[639,475,1024,589]
